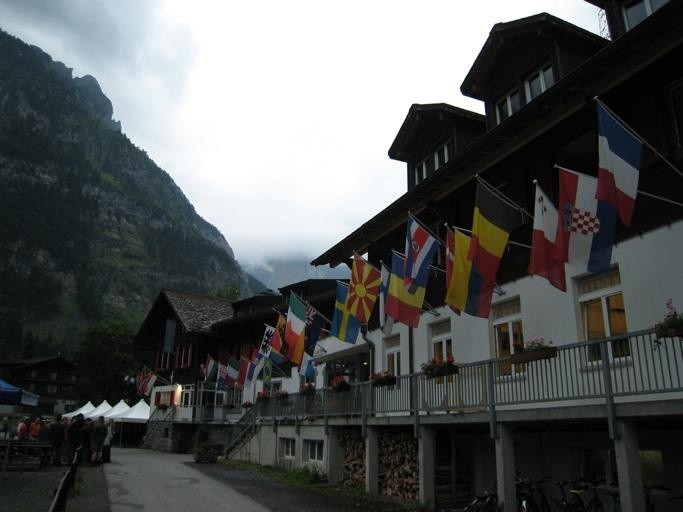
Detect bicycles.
[461,469,662,512]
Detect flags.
[444,206,509,318]
[136,364,158,397]
[204,356,256,389]
[329,216,442,344]
[247,293,325,385]
[529,170,617,292]
[594,105,642,226]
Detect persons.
[0,414,116,465]
